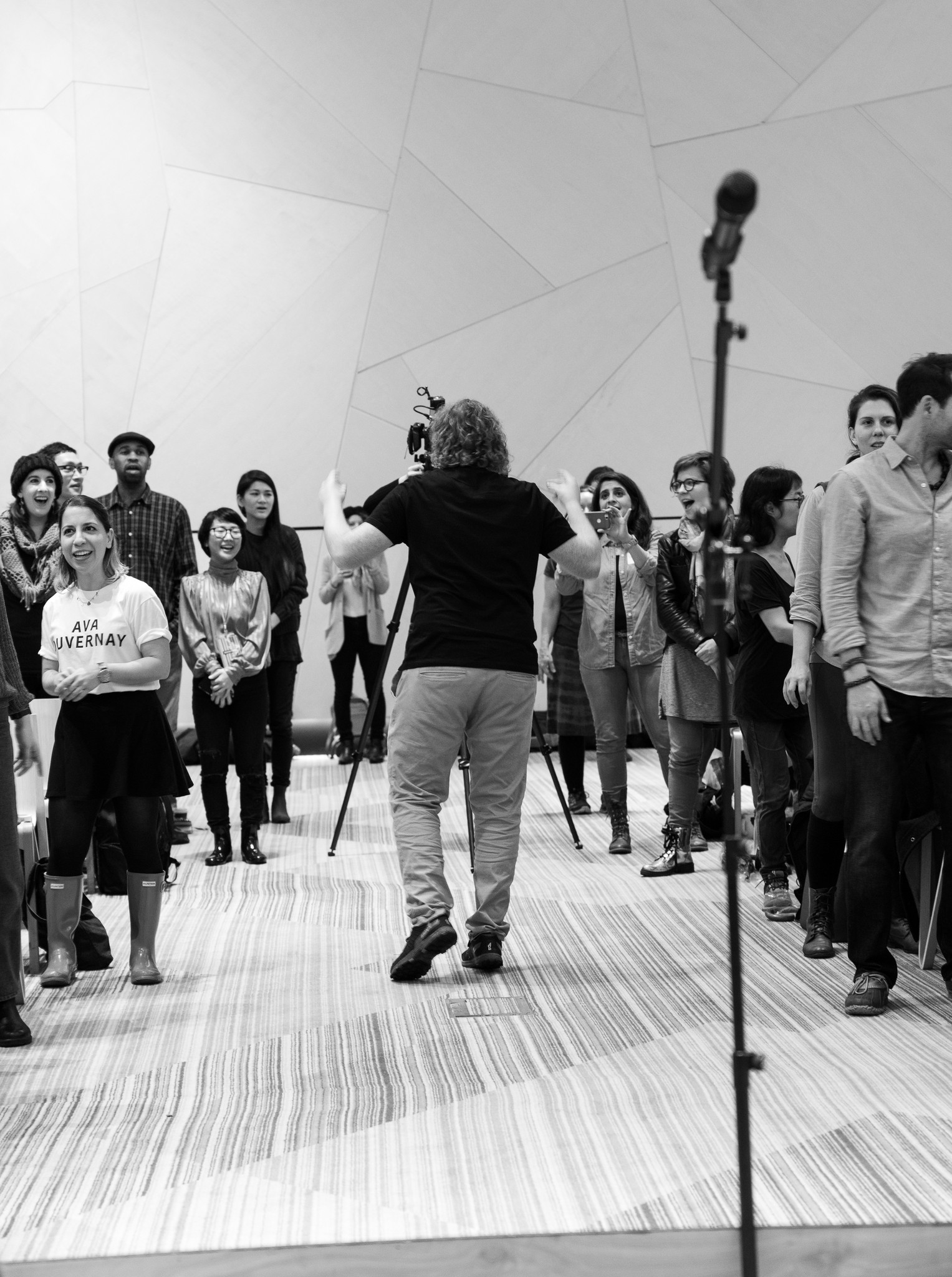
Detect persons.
[0,351,951,1065]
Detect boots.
[888,896,938,954]
[240,823,268,864]
[204,823,232,866]
[124,868,164,985]
[803,886,835,958]
[567,790,709,875]
[272,786,291,822]
[40,873,87,987]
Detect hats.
[10,453,63,502]
[108,432,154,457]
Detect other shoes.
[339,739,353,764]
[369,738,385,763]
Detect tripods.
[327,478,586,876]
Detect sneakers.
[844,972,891,1015]
[763,870,792,907]
[460,934,506,970]
[388,914,457,982]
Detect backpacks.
[93,791,179,896]
[23,856,114,971]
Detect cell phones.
[585,511,610,530]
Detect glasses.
[670,478,709,493]
[777,495,805,508]
[208,526,244,539]
[55,465,91,475]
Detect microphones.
[703,167,758,278]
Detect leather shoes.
[0,998,33,1047]
